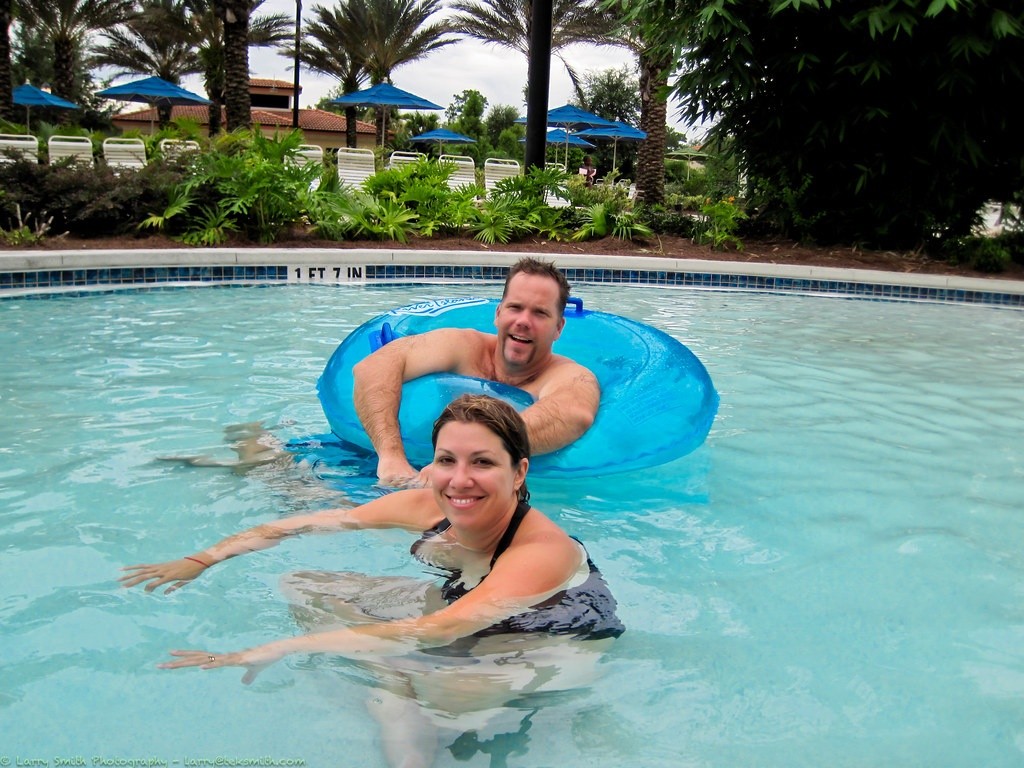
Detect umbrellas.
[327,77,445,164]
[94,76,213,137]
[11,79,81,141]
[664,145,719,182]
[513,100,648,188]
[409,125,476,158]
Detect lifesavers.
[313,296,721,482]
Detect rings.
[208,655,216,663]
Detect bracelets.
[184,556,210,567]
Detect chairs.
[337,147,377,223]
[102,137,148,179]
[48,135,95,170]
[161,139,206,185]
[0,133,39,169]
[544,163,571,209]
[283,145,324,193]
[439,154,477,227]
[389,151,428,171]
[484,158,521,199]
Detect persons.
[351,257,599,488]
[353,393,580,619]
[580,155,594,188]
